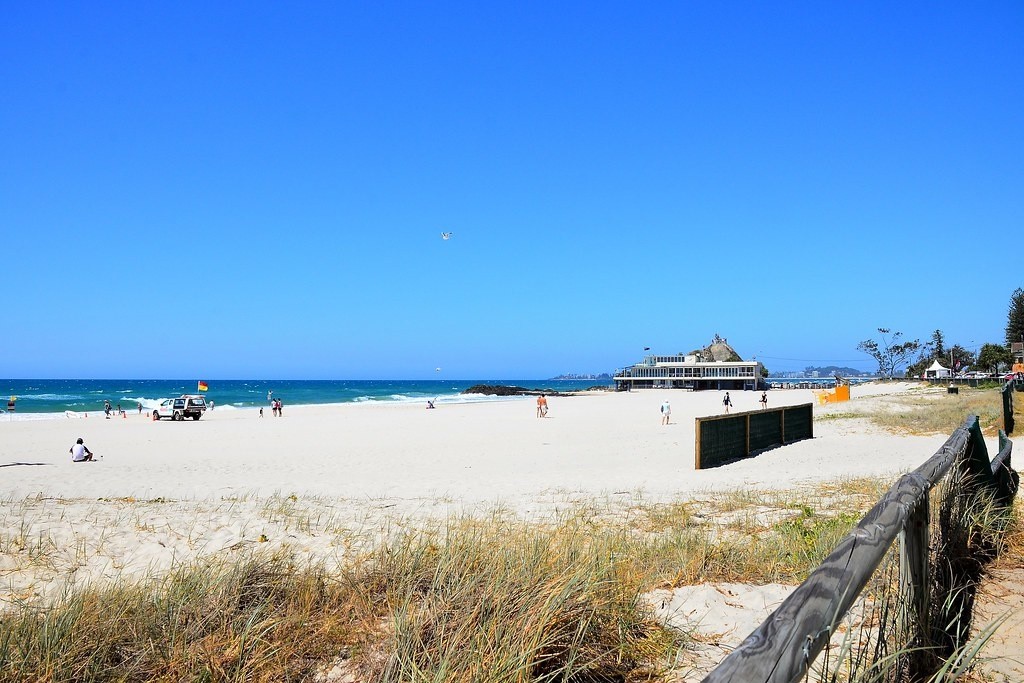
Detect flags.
[199,382,208,391]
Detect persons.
[922,373,925,380]
[209,399,215,411]
[137,401,143,414]
[761,392,768,410]
[950,381,954,388]
[428,401,435,409]
[722,392,732,415]
[889,375,892,382]
[258,406,264,418]
[104,400,112,416]
[884,371,887,382]
[952,369,994,381]
[267,391,273,401]
[277,398,282,417]
[536,393,549,418]
[69,438,94,463]
[271,398,278,417]
[661,399,671,425]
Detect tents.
[925,359,948,378]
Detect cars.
[962,370,1024,380]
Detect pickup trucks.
[151,397,206,422]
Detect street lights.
[938,342,954,368]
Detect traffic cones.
[145,411,149,417]
[152,414,156,422]
[84,412,88,418]
[112,411,115,416]
[123,412,126,418]
